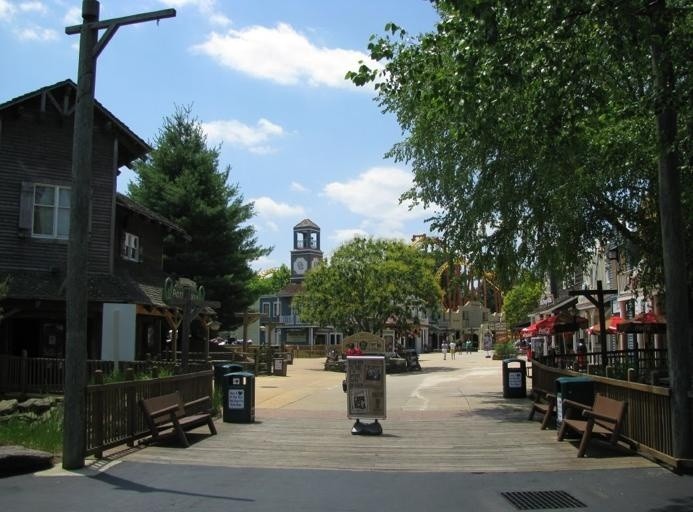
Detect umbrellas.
[520,314,588,367]
[617,312,666,365]
[584,316,640,370]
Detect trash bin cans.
[554,376,595,438]
[213,365,243,394]
[273,359,287,375]
[220,364,256,423]
[502,358,527,399]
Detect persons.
[345,343,364,356]
[441,339,472,360]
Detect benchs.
[528,386,557,430]
[140,391,217,447]
[557,393,628,457]
